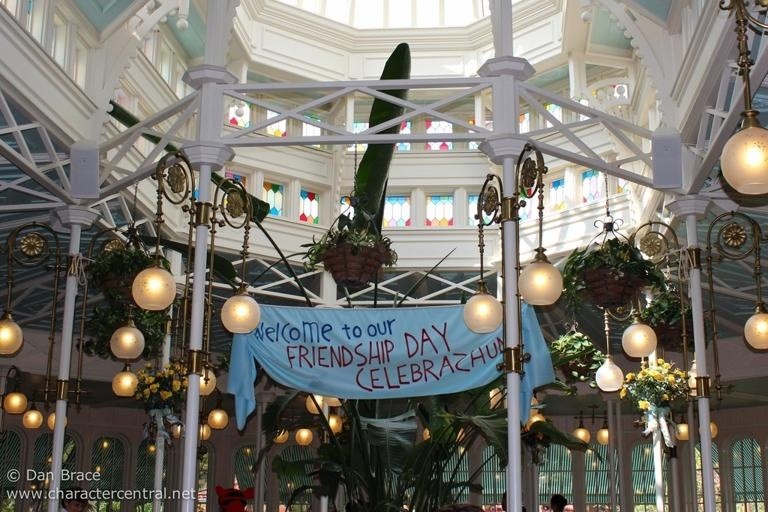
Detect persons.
[60,486,91,512]
[550,493,568,511]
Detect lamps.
[0,144,767,444]
[712,1,766,197]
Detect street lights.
[111,283,217,512]
[621,211,768,512]
[594,279,712,512]
[131,142,277,511]
[462,139,564,512]
[0,204,147,512]
